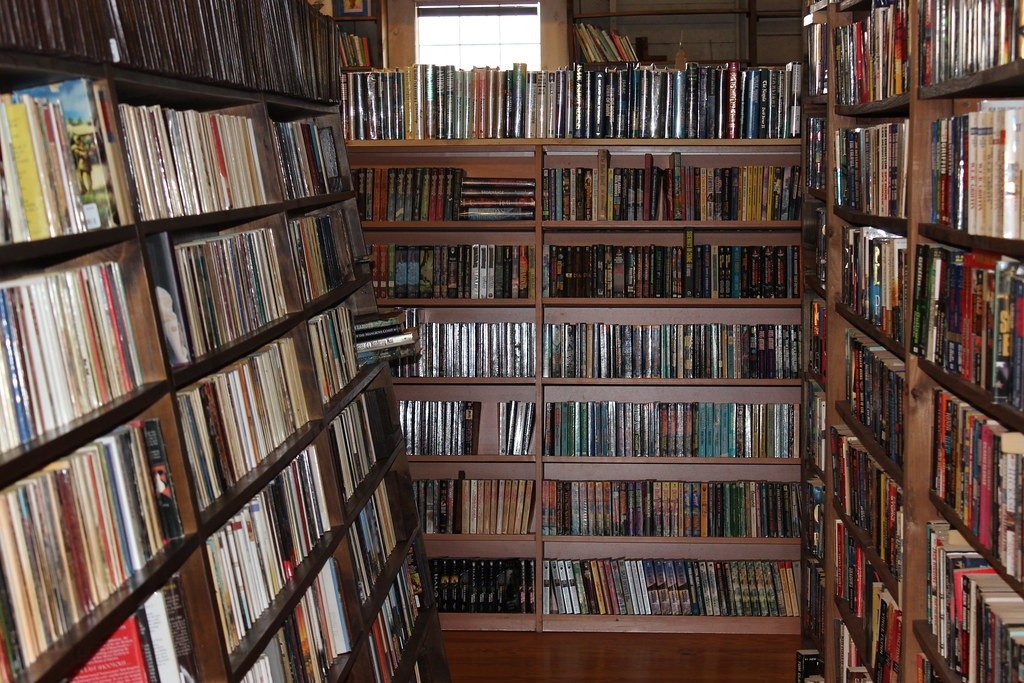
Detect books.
[0,0,1024,683]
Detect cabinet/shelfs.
[0,0,1024,683]
